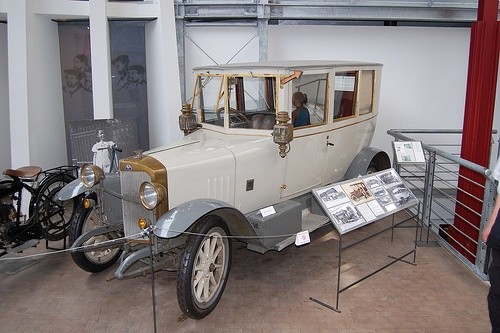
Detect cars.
[57,59,393,321]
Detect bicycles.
[0,165,81,258]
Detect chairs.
[247,115,276,131]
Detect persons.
[484,151,499,332]
[91,129,118,173]
[291,92,312,127]
